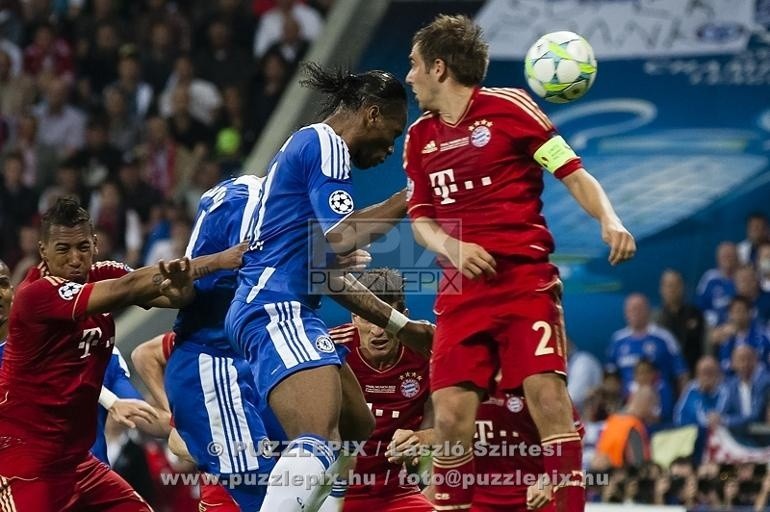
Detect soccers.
[525,30,597,104]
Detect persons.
[2,0,769,512]
